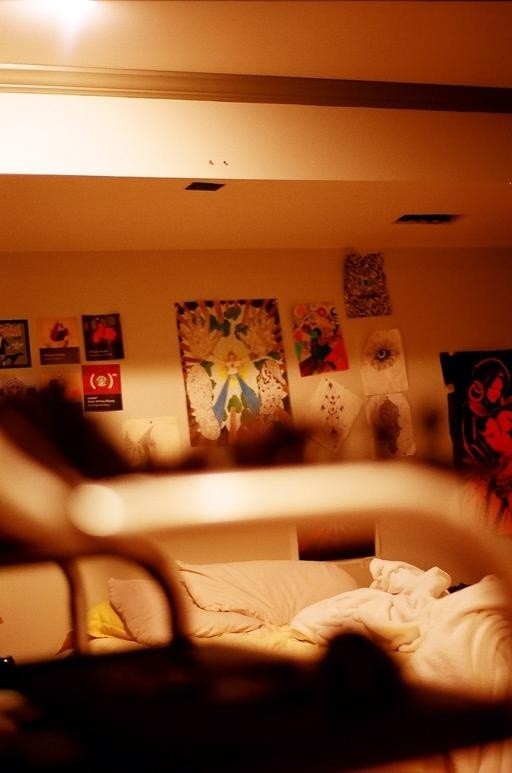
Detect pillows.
[87,599,135,642]
[107,579,265,647]
[175,559,359,626]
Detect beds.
[71,557,512,773]
